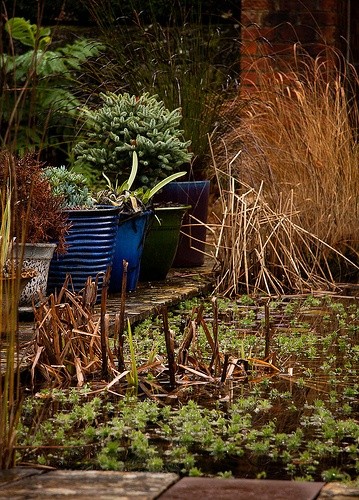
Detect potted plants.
[39,165,120,308]
[0,179,37,336]
[75,90,191,282]
[92,150,187,296]
[0,148,75,314]
[43,35,253,269]
